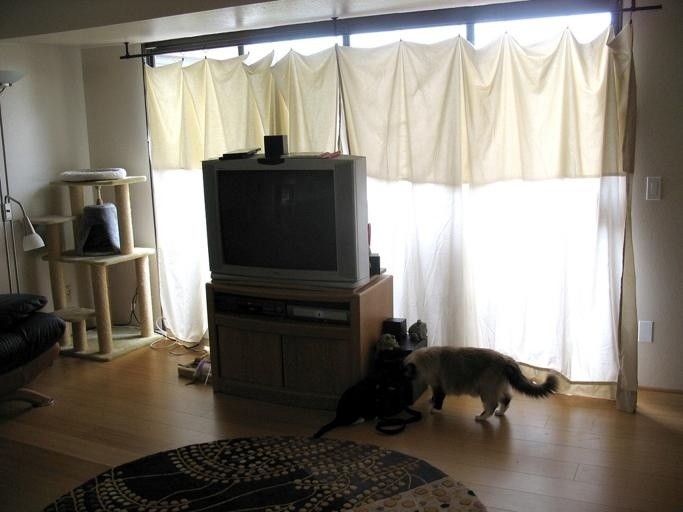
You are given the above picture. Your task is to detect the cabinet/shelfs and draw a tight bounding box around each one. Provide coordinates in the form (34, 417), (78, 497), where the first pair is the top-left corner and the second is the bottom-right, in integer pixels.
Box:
(204, 274), (392, 410)
(23, 169), (159, 360)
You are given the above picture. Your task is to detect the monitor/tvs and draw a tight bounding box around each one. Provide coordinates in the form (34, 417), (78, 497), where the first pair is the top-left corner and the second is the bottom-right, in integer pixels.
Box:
(202, 152), (370, 288)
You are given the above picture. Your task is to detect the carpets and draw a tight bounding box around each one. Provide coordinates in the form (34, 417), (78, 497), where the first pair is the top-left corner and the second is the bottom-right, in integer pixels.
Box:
(41, 435), (491, 512)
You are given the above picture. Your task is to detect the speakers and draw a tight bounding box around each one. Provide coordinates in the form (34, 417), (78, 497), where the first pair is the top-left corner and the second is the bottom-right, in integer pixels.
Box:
(370, 253), (380, 277)
(264, 135), (287, 156)
(384, 317), (407, 341)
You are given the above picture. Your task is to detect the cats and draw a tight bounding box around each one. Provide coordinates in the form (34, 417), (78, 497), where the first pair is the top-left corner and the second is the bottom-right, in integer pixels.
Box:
(313, 348), (414, 439)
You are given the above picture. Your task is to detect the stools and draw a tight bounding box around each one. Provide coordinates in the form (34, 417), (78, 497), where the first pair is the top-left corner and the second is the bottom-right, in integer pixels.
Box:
(0, 293), (65, 409)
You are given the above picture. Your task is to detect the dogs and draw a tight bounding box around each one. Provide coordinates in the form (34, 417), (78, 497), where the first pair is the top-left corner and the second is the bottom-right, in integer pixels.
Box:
(402, 346), (559, 421)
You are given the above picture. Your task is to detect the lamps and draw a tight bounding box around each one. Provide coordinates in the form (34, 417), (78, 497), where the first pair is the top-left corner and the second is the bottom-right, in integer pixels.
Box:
(0, 70), (46, 295)
